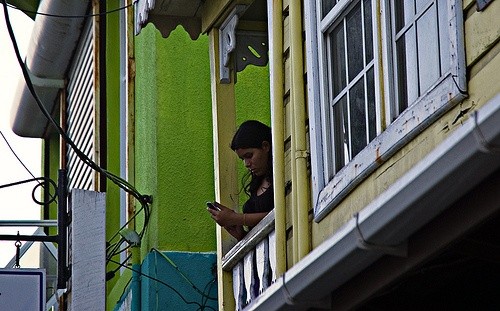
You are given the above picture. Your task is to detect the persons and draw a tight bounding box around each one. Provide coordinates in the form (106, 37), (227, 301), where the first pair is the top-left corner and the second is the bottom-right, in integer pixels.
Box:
(208, 119), (275, 240)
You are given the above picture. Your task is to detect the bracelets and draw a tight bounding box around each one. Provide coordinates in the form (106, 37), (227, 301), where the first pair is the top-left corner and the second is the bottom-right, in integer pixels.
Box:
(244, 213), (246, 226)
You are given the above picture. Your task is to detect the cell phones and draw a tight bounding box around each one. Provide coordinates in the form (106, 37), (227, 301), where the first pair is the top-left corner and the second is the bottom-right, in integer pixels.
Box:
(207, 202), (219, 211)
